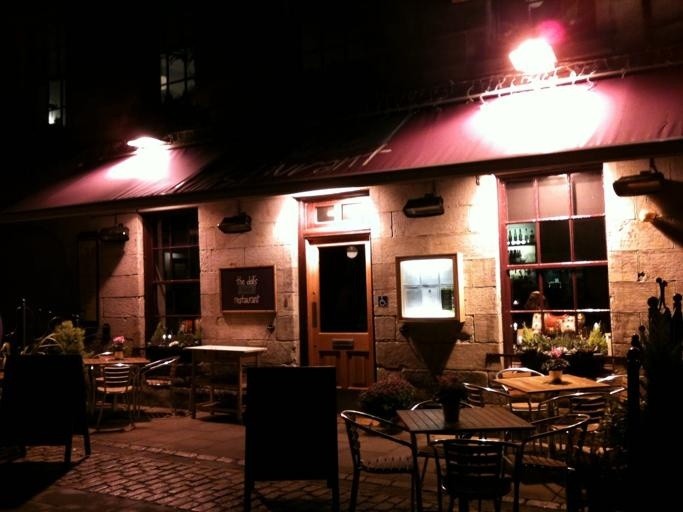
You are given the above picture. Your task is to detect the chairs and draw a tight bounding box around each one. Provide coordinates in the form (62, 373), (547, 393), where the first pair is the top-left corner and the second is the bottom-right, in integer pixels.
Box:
(81, 356), (180, 430)
(0, 354), (90, 462)
(244, 365), (339, 512)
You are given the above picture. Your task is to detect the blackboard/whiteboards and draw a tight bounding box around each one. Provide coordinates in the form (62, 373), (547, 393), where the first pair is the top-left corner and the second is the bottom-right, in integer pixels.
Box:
(218, 264), (278, 314)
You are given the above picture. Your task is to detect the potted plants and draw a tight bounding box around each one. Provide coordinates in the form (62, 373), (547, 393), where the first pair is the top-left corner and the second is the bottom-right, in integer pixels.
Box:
(519, 321), (607, 379)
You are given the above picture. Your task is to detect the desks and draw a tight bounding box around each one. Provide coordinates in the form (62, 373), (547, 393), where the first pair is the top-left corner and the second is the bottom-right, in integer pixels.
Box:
(183, 344), (268, 422)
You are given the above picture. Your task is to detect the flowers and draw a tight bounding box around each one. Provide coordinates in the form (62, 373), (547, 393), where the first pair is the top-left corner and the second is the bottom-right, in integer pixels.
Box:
(541, 347), (569, 370)
(112, 336), (128, 351)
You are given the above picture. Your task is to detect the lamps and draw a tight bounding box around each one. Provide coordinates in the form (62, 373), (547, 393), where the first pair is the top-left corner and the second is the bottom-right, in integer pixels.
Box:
(613, 174), (663, 197)
(99, 223), (129, 243)
(218, 213), (252, 233)
(403, 192), (444, 218)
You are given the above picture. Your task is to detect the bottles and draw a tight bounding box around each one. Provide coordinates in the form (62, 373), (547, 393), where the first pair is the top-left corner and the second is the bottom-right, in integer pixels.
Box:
(508, 249), (521, 264)
(508, 228), (535, 245)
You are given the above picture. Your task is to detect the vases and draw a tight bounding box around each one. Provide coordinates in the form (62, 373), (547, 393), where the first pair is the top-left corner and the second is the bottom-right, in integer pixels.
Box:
(115, 351), (124, 359)
